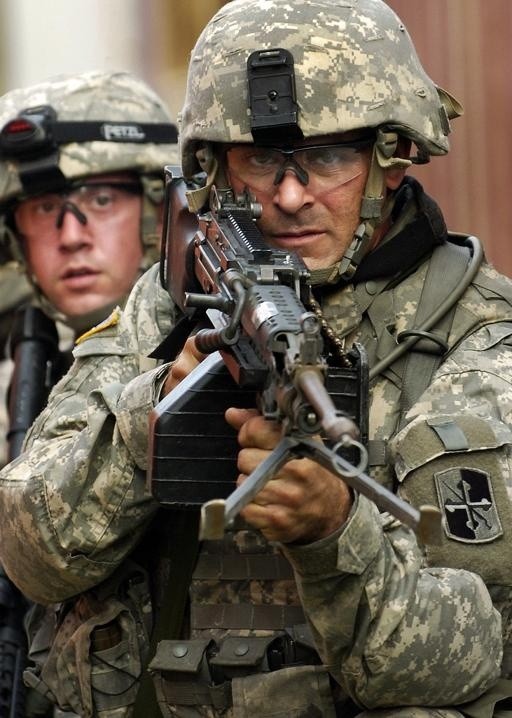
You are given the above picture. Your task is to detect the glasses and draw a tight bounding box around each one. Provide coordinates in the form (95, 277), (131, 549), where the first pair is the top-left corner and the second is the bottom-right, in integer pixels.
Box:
(225, 143), (373, 200)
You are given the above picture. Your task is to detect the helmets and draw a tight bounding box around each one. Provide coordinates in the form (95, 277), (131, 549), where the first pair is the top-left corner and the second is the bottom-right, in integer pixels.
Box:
(181, 0), (451, 181)
(14, 180), (139, 239)
(1, 67), (180, 206)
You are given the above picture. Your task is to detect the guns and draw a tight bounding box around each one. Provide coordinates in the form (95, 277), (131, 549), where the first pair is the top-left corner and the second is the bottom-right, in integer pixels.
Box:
(145, 164), (442, 547)
(6, 304), (59, 463)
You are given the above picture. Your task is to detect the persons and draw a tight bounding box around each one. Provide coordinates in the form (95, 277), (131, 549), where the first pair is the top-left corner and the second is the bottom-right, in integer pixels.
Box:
(1, 67), (181, 714)
(1, 0), (512, 715)
